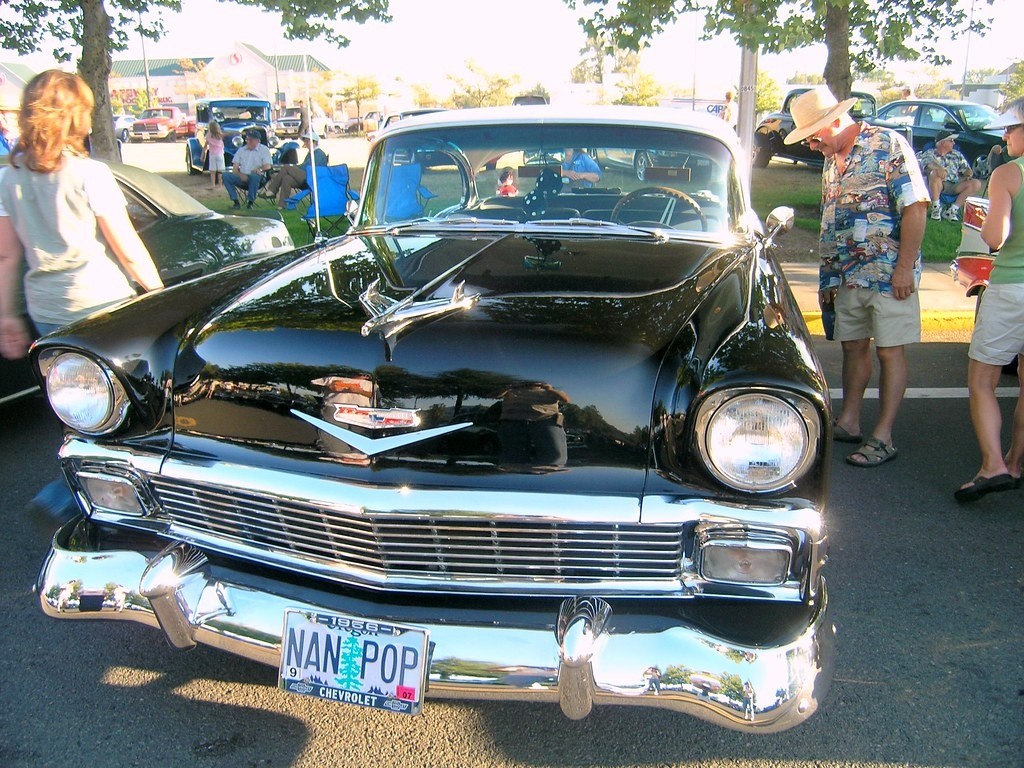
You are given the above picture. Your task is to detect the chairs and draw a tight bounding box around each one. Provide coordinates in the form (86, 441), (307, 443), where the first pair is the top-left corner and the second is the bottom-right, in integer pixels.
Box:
(462, 208), (580, 223)
(924, 141), (971, 221)
(234, 153), (360, 245)
(377, 161), (438, 222)
(584, 210), (715, 225)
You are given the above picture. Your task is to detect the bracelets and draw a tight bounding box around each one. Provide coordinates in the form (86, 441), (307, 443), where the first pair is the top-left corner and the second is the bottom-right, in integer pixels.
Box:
(258, 168), (263, 172)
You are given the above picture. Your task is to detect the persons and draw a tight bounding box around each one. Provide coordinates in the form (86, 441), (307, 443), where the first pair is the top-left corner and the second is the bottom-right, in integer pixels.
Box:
(258, 131), (327, 210)
(311, 372), (378, 464)
(496, 166), (519, 196)
(221, 129), (273, 209)
(955, 97), (1024, 498)
(500, 381), (569, 472)
(921, 130), (981, 222)
(901, 84), (918, 113)
(0, 69), (165, 523)
(724, 92), (738, 132)
(784, 87), (931, 466)
(200, 122), (225, 190)
(561, 148), (602, 188)
(297, 97), (318, 148)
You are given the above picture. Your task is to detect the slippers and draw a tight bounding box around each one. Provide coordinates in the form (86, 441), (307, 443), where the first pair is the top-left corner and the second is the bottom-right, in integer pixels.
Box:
(955, 473), (1024, 501)
(832, 418), (864, 444)
(847, 436), (898, 466)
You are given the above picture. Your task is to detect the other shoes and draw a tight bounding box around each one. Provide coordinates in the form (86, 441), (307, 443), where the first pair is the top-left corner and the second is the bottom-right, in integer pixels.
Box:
(247, 203), (253, 209)
(258, 193), (276, 198)
(230, 203), (241, 210)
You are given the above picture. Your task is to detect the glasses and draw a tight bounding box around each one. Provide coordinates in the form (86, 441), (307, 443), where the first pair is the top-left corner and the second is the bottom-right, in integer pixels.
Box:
(945, 138), (954, 142)
(1004, 124), (1024, 134)
(800, 129), (823, 147)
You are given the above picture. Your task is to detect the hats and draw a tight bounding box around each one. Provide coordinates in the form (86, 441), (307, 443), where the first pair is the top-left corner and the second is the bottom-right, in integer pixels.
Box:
(983, 110), (1024, 129)
(934, 131), (959, 145)
(783, 86), (858, 145)
(301, 132), (319, 142)
(245, 129), (262, 139)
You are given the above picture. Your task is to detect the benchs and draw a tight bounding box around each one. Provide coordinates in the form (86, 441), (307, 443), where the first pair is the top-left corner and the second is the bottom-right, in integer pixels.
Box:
(486, 195), (718, 218)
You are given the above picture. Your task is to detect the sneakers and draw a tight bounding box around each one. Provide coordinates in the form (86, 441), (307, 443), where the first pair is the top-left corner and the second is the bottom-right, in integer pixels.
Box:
(941, 208), (958, 224)
(931, 205), (943, 220)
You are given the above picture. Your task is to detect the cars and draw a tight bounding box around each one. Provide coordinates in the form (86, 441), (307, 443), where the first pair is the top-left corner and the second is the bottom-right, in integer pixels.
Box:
(271, 106), (503, 171)
(876, 97), (1024, 180)
(751, 83), (876, 172)
(0, 158), (296, 289)
(114, 102), (196, 142)
(185, 99), (276, 180)
(25, 107), (835, 735)
(585, 146), (716, 182)
